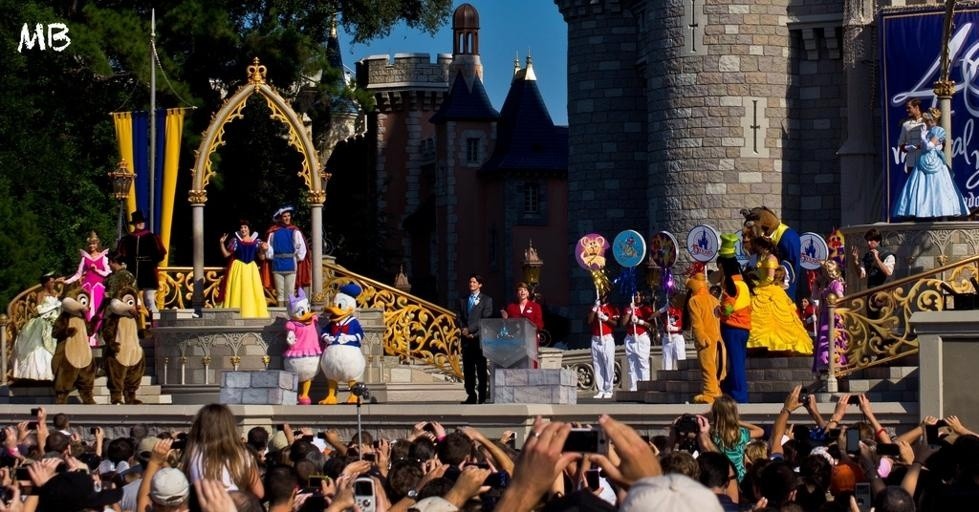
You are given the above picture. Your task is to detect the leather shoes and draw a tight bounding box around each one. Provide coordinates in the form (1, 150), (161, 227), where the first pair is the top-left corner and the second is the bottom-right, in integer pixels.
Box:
(461, 397), (485, 404)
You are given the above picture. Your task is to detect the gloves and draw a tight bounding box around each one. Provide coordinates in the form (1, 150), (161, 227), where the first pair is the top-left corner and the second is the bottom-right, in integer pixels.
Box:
(632, 315), (643, 326)
(597, 311), (608, 322)
(659, 303), (670, 313)
(626, 302), (635, 314)
(905, 145), (917, 151)
(920, 124), (929, 139)
(667, 324), (678, 332)
(592, 299), (601, 311)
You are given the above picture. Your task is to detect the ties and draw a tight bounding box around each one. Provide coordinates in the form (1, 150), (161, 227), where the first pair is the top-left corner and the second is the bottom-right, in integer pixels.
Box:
(468, 297), (475, 312)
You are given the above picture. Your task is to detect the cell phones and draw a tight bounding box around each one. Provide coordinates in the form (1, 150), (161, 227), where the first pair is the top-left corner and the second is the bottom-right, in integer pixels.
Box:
(585, 470), (600, 491)
(510, 432), (517, 440)
(561, 422), (610, 457)
(876, 443), (900, 456)
(847, 395), (860, 404)
(485, 471), (508, 488)
(846, 427), (861, 456)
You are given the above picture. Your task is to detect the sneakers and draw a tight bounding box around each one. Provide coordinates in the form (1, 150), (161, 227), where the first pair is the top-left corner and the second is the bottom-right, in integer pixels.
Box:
(593, 391), (613, 399)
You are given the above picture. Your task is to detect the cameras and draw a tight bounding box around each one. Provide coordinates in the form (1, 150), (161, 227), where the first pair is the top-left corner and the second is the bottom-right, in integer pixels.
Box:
(855, 482), (871, 512)
(863, 252), (875, 262)
(680, 414), (703, 434)
(799, 388), (808, 405)
(351, 382), (369, 399)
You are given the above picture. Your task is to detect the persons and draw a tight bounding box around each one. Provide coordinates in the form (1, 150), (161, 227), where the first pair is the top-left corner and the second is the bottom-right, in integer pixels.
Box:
(622, 289), (656, 391)
(220, 217), (273, 321)
(892, 107), (971, 221)
(97, 284), (148, 405)
(455, 273), (494, 404)
(586, 286), (622, 399)
(117, 210), (167, 330)
(812, 257), (851, 372)
(651, 290), (686, 371)
(59, 231), (112, 349)
(13, 267), (63, 383)
(897, 98), (943, 174)
(798, 296), (817, 340)
(500, 282), (544, 370)
(264, 205), (307, 307)
(52, 288), (101, 404)
(0, 381), (978, 512)
(746, 235), (812, 354)
(850, 228), (902, 361)
(95, 249), (137, 336)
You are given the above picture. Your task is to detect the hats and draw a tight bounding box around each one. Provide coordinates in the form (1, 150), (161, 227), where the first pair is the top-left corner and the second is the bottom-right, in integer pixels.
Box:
(621, 474), (725, 512)
(40, 471), (123, 512)
(150, 468), (188, 506)
(136, 437), (162, 460)
(830, 464), (866, 495)
